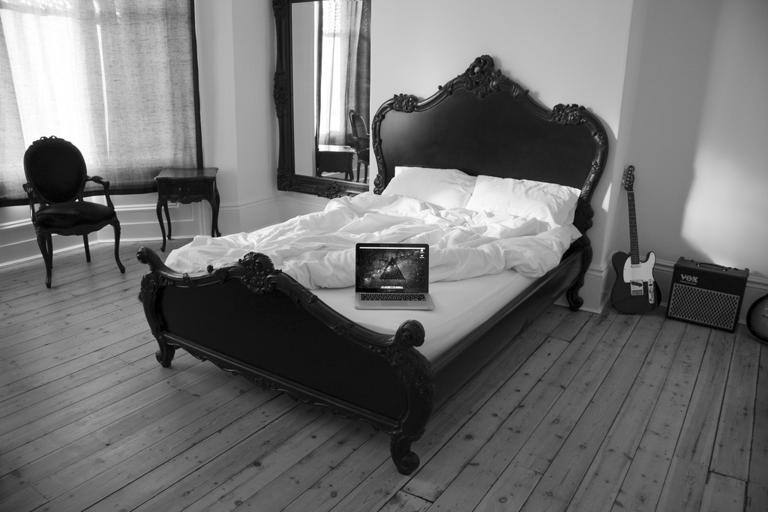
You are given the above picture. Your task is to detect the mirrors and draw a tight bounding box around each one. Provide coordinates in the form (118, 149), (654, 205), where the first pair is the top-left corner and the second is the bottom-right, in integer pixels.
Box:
(269, 1), (375, 199)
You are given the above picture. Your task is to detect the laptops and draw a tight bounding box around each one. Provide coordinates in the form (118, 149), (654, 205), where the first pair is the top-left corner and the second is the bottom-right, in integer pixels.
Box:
(355, 243), (435, 311)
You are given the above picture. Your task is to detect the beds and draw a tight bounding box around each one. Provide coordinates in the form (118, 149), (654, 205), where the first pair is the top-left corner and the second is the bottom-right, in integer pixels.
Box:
(132, 51), (609, 477)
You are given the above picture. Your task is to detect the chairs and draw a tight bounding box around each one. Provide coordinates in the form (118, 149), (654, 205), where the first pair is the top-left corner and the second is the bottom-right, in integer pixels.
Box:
(21, 135), (127, 289)
(347, 107), (371, 185)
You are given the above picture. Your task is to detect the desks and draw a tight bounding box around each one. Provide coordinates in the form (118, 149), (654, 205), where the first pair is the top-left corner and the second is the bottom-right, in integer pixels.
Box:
(154, 165), (223, 252)
(314, 143), (355, 184)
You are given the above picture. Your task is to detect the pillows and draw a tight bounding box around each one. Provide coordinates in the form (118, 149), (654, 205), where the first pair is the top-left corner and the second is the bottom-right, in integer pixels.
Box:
(462, 171), (582, 240)
(376, 163), (477, 214)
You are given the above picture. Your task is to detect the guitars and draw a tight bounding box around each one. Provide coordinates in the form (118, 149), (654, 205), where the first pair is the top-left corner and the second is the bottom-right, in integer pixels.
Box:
(611, 165), (662, 314)
